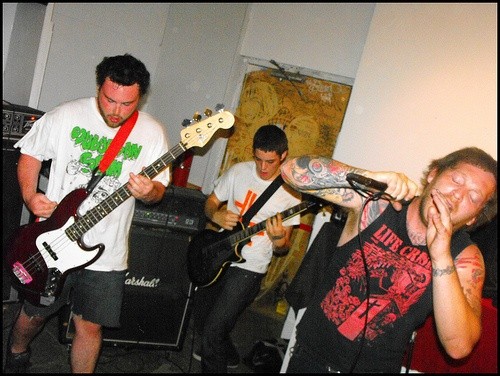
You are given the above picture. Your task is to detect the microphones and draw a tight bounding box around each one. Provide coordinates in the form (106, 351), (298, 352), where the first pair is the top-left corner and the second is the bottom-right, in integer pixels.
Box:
(347, 173), (412, 205)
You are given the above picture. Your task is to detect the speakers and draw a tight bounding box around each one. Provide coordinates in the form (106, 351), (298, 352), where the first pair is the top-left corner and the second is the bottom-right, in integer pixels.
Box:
(0, 137), (24, 301)
(58, 222), (199, 352)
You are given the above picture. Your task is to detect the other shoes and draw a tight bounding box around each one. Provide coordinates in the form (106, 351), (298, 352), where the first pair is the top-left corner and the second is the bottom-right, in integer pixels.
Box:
(9, 349), (30, 376)
(225, 344), (238, 369)
(193, 340), (202, 360)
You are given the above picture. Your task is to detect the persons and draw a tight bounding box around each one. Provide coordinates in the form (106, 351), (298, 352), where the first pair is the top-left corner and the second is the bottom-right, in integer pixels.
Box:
(190, 125), (299, 373)
(4, 54), (172, 373)
(279, 146), (496, 373)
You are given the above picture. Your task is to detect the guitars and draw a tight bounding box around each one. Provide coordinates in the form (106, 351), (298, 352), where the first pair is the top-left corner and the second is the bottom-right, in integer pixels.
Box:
(187, 197), (320, 288)
(2, 103), (236, 319)
